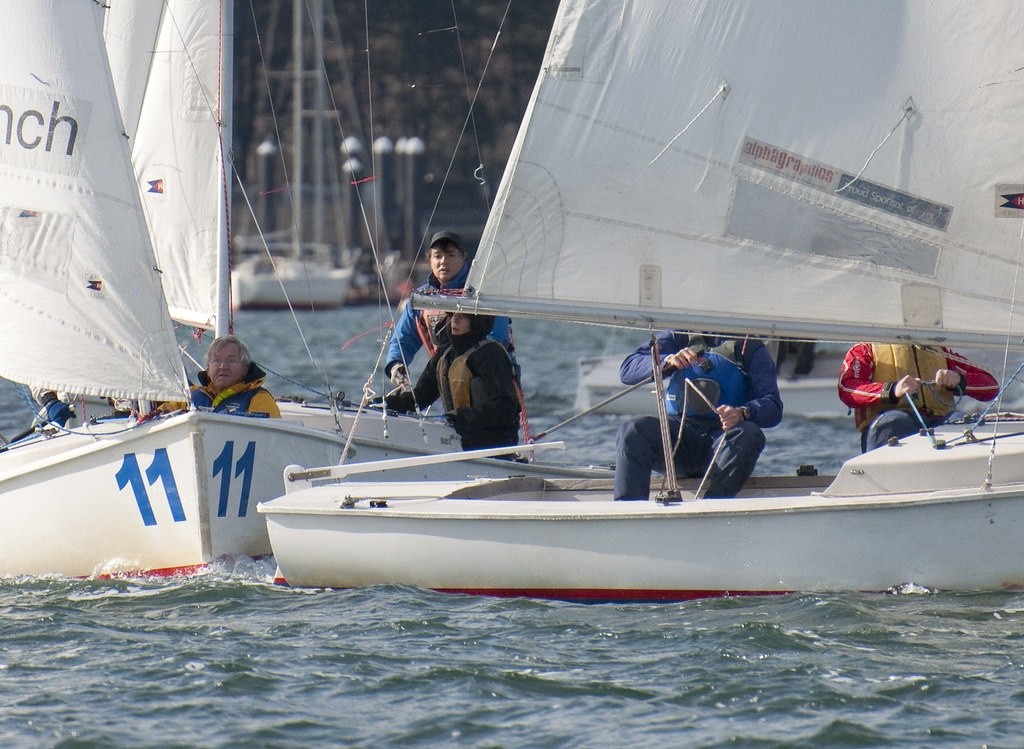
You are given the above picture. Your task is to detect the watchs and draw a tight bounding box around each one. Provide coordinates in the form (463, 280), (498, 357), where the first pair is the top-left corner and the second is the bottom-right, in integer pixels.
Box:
(740, 406), (750, 421)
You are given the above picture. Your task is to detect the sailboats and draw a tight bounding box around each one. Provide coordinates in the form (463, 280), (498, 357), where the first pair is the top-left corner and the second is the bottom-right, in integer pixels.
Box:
(231, 3), (363, 314)
(103, 0), (612, 477)
(248, 1), (1024, 603)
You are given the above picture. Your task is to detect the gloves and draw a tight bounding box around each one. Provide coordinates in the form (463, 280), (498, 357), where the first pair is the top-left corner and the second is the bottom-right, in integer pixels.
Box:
(41, 392), (77, 427)
(370, 395), (397, 410)
(443, 407), (465, 426)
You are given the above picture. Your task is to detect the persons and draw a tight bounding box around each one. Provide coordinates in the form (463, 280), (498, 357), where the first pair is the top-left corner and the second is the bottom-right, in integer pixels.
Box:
(838, 342), (1000, 454)
(614, 329), (783, 501)
(154, 335), (281, 419)
(384, 230), (520, 392)
(30, 385), (117, 431)
(366, 310), (522, 462)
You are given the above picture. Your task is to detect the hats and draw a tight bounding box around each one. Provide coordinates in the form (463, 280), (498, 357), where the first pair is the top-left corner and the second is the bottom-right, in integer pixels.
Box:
(428, 231), (463, 257)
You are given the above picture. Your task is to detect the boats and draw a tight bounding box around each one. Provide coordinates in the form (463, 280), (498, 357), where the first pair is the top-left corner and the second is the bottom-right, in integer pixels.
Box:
(0, 1), (468, 598)
(570, 345), (870, 424)
